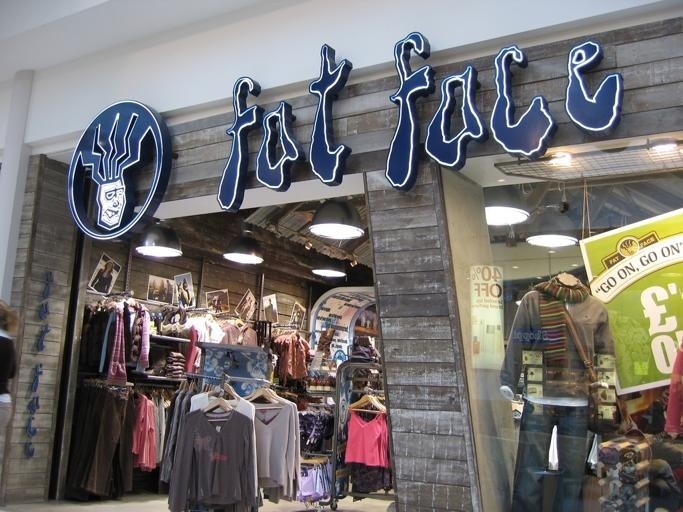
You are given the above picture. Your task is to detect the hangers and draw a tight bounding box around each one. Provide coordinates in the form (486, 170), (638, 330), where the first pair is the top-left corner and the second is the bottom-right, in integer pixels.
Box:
(84, 372), (281, 413)
(215, 313), (300, 335)
(348, 388), (386, 414)
(301, 452), (330, 470)
(97, 294), (209, 317)
(304, 402), (331, 413)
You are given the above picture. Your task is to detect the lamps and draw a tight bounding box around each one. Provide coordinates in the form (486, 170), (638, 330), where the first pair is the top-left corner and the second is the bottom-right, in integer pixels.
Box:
(485, 186), (530, 225)
(222, 223), (265, 264)
(526, 204), (578, 247)
(312, 256), (347, 277)
(308, 200), (365, 240)
(135, 218), (183, 258)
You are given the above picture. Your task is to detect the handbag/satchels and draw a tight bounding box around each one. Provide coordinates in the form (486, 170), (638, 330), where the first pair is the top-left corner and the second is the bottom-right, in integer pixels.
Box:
(588, 381), (622, 434)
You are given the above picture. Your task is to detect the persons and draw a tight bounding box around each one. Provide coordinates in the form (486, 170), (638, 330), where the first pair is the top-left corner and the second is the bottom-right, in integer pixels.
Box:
(499, 271), (618, 512)
(586, 384), (683, 511)
(0, 302), (21, 474)
